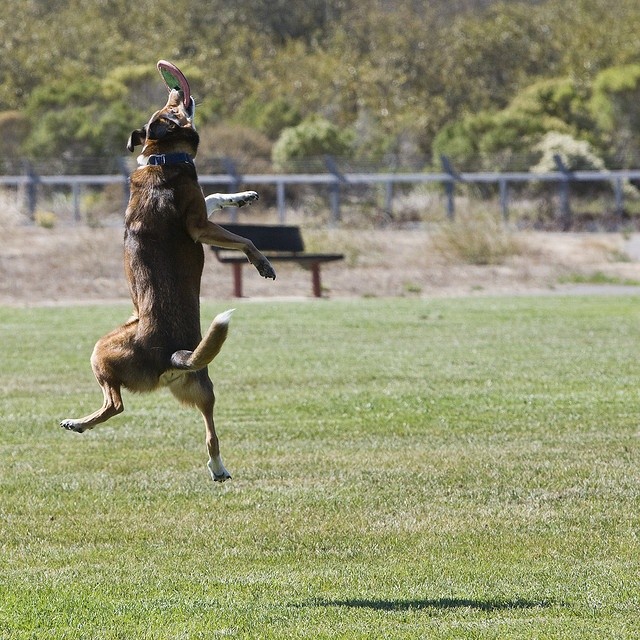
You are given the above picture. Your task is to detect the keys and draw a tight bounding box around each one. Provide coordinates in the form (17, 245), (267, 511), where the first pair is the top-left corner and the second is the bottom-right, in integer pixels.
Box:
(156, 60), (190, 109)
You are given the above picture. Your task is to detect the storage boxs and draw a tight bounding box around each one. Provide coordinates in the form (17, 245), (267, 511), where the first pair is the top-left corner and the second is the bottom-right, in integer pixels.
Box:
(208, 222), (347, 299)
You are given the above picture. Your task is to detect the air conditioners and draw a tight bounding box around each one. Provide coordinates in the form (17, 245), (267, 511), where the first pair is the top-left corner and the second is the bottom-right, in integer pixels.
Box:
(60, 85), (276, 481)
